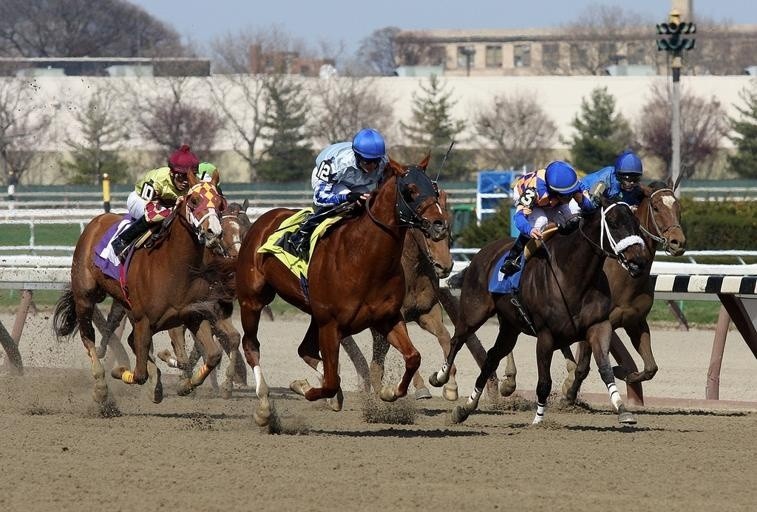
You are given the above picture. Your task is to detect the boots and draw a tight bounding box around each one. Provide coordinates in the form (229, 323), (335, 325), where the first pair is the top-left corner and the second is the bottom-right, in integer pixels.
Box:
(111, 215), (155, 265)
(500, 233), (531, 275)
(290, 206), (332, 263)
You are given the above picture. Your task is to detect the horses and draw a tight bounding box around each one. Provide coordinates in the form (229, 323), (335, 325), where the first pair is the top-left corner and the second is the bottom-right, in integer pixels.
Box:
(54, 167), (229, 404)
(235, 148), (451, 428)
(428, 185), (650, 427)
(100, 198), (247, 401)
(341, 187), (458, 401)
(500, 177), (687, 397)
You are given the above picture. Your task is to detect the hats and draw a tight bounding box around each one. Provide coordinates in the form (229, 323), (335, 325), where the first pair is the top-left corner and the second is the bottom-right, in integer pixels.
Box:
(545, 161), (580, 194)
(352, 128), (386, 158)
(169, 145), (199, 171)
(614, 152), (642, 175)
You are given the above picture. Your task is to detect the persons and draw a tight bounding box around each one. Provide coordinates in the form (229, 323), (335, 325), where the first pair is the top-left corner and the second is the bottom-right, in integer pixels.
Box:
(569, 150), (642, 220)
(500, 161), (596, 274)
(288, 128), (396, 262)
(110, 145), (199, 264)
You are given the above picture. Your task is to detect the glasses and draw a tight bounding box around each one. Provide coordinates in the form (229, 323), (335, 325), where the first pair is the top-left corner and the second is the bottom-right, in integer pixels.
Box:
(619, 174), (641, 182)
(360, 158), (381, 164)
(174, 173), (188, 182)
